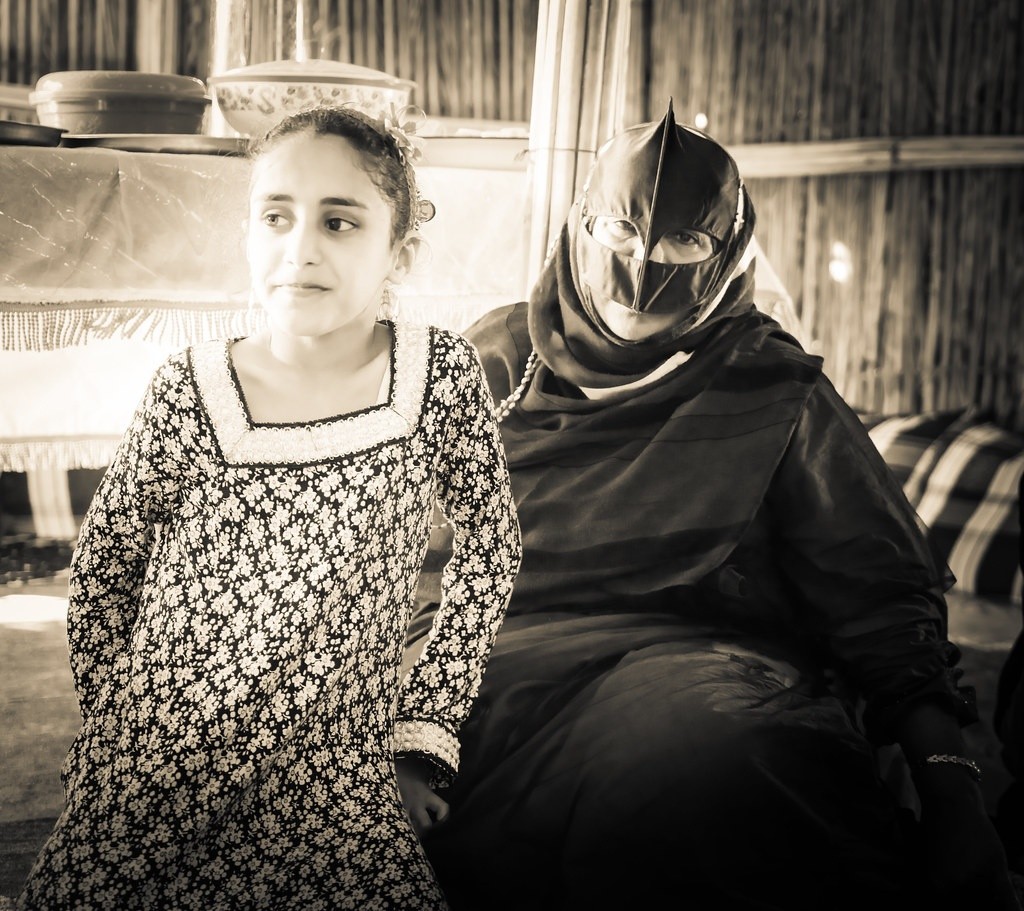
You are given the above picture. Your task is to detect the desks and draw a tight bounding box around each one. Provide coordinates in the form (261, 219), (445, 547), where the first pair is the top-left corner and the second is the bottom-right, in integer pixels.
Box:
(0, 139), (532, 545)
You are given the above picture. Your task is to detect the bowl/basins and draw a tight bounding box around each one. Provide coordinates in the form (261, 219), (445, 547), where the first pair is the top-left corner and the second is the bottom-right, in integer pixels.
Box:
(28, 71), (212, 135)
(206, 58), (418, 138)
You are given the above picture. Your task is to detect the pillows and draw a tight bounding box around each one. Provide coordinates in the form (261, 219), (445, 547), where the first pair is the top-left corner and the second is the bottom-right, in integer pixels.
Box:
(855, 407), (1024, 609)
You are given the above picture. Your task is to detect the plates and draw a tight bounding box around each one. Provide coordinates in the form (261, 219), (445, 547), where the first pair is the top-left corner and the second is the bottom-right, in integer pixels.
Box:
(0, 119), (69, 148)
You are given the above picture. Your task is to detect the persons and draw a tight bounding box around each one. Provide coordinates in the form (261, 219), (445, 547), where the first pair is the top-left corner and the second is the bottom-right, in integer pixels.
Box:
(401, 95), (1024, 911)
(12, 106), (524, 911)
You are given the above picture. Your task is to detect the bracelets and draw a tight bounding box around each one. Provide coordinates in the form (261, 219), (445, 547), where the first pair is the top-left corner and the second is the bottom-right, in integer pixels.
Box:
(926, 754), (984, 784)
